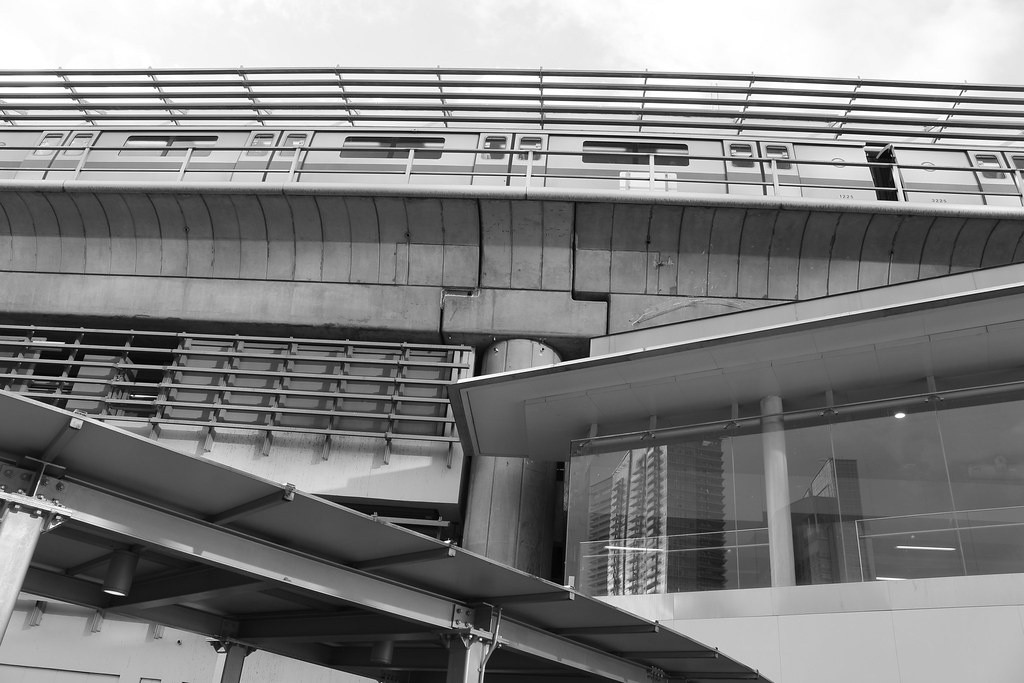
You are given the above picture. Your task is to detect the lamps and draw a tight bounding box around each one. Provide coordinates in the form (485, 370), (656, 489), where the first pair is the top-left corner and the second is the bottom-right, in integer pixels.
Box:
(101, 548), (139, 597)
(370, 640), (394, 664)
